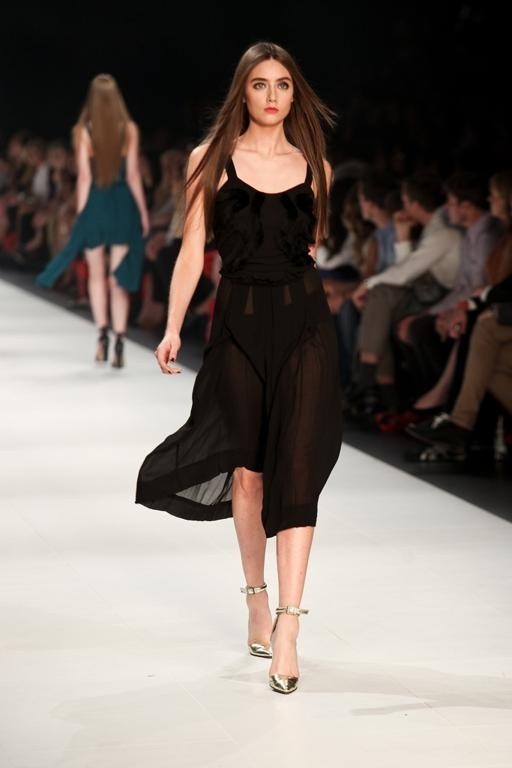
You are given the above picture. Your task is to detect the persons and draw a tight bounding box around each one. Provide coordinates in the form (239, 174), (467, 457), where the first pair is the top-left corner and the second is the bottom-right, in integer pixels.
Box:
(1, 128), (225, 340)
(312, 156), (510, 467)
(155, 40), (346, 696)
(70, 72), (152, 368)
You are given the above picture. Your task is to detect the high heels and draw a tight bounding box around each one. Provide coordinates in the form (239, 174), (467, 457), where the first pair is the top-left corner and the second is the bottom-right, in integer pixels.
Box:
(240, 584), (309, 694)
(96, 326), (126, 367)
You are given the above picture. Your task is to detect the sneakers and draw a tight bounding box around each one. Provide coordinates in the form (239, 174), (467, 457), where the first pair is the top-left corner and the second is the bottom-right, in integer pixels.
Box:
(344, 361), (473, 464)
